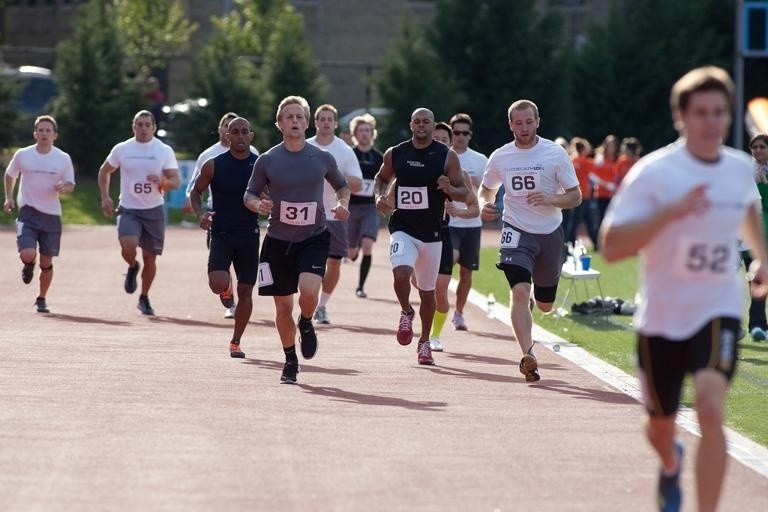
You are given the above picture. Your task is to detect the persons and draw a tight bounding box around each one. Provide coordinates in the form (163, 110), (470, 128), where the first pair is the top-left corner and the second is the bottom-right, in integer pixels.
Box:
(384, 122), (480, 351)
(97, 109), (182, 316)
(596, 63), (768, 512)
(338, 127), (355, 149)
(3, 115), (76, 314)
(447, 112), (489, 331)
(243, 95), (351, 384)
(304, 103), (366, 324)
(735, 134), (768, 343)
(185, 111), (259, 318)
(587, 135), (620, 228)
(372, 107), (470, 366)
(477, 99), (583, 382)
(188, 116), (270, 359)
(144, 76), (165, 138)
(613, 137), (642, 193)
(345, 113), (385, 299)
(563, 137), (617, 255)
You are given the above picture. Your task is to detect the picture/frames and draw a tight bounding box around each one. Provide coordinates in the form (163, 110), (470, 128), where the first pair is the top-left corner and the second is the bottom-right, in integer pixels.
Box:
(556, 261), (609, 323)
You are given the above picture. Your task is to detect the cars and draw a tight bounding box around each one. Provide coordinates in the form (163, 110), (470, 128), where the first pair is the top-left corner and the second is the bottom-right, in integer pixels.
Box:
(580, 255), (591, 271)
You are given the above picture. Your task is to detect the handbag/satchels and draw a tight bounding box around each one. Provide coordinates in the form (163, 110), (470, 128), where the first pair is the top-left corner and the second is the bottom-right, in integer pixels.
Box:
(336, 198), (349, 205)
(159, 177), (167, 191)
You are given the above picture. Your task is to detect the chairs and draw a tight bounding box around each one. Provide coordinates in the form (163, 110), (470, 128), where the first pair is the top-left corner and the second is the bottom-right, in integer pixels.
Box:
(224, 304), (236, 318)
(280, 359), (299, 384)
(520, 352), (540, 382)
(125, 261), (140, 293)
(417, 341), (434, 364)
(137, 295), (153, 314)
(751, 327), (766, 342)
(220, 289), (234, 308)
(36, 297), (49, 312)
(429, 338), (443, 351)
(397, 305), (415, 346)
(298, 315), (319, 360)
(22, 263), (35, 284)
(452, 312), (467, 330)
(230, 338), (245, 358)
(314, 306), (329, 323)
(356, 289), (366, 298)
(657, 443), (682, 511)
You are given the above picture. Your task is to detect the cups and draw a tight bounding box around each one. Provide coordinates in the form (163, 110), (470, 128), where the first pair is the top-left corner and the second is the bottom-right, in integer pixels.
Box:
(453, 130), (472, 136)
